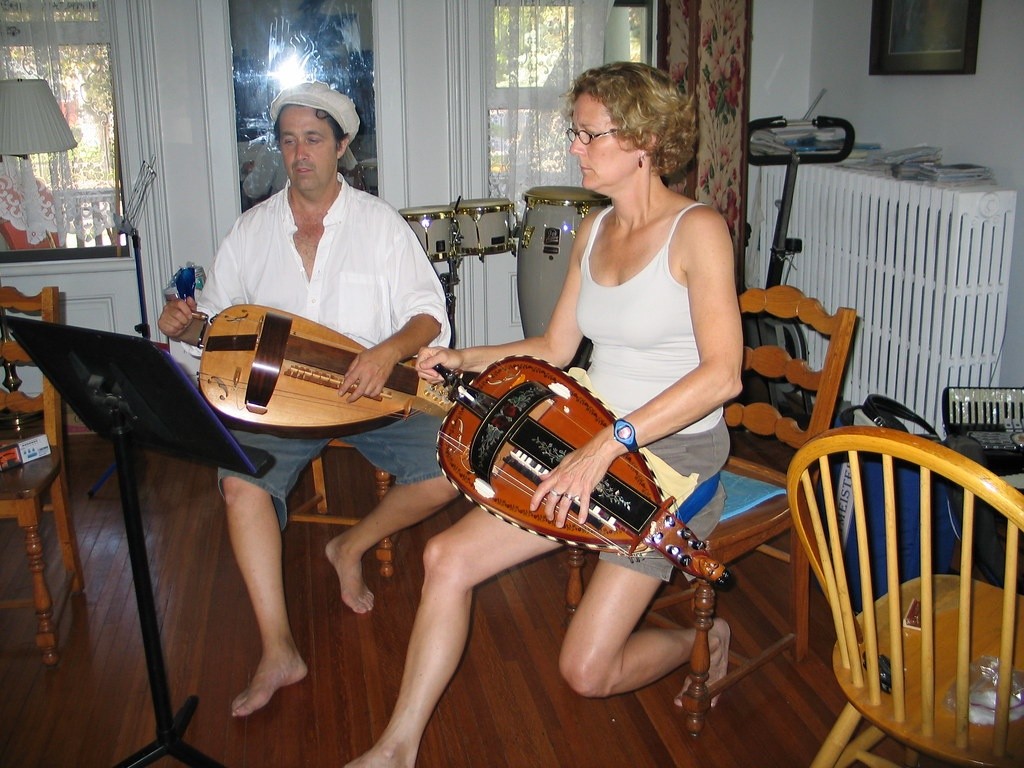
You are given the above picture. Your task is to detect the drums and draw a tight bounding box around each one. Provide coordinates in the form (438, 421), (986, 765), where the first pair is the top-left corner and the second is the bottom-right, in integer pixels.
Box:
(516, 185), (613, 339)
(398, 197), (512, 263)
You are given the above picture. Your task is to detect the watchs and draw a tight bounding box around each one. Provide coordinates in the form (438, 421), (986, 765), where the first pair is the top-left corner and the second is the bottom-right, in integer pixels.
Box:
(613, 418), (639, 452)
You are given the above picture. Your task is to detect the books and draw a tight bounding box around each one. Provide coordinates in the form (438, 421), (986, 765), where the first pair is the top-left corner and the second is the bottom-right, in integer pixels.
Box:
(752, 118), (989, 183)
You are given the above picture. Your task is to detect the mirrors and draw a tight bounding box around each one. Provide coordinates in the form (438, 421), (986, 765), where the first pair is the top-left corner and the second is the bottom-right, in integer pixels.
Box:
(196, 0), (409, 256)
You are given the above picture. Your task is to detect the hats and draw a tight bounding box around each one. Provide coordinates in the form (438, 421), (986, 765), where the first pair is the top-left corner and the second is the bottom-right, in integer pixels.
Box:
(269, 80), (361, 147)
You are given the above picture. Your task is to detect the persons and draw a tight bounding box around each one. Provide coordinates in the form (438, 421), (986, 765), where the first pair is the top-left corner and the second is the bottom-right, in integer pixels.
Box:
(342, 61), (742, 767)
(158, 80), (461, 716)
(239, 128), (360, 198)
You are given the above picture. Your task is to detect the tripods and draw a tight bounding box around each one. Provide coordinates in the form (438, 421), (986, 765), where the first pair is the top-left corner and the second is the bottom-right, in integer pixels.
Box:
(0, 309), (274, 768)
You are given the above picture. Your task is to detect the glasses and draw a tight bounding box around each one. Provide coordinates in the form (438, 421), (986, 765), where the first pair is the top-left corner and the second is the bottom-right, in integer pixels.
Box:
(566, 127), (616, 144)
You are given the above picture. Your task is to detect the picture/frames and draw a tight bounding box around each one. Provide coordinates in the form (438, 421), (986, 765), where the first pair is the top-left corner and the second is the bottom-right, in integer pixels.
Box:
(869, 0), (983, 75)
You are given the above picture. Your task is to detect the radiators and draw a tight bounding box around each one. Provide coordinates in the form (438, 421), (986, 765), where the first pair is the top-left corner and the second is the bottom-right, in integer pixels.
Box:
(758, 164), (1017, 443)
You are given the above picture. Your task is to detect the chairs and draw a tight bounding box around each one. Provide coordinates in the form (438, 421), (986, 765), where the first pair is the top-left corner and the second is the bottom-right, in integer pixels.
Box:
(0, 285), (84, 664)
(564, 287), (855, 738)
(787, 427), (1024, 768)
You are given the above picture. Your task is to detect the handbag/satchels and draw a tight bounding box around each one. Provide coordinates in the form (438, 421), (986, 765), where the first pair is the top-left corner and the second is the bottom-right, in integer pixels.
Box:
(809, 391), (962, 613)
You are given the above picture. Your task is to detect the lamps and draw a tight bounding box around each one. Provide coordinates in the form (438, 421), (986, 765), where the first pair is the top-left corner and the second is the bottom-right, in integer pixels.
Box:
(0, 78), (77, 243)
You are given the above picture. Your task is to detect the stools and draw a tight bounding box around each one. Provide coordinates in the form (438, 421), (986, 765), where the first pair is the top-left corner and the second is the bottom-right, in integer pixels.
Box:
(287, 439), (399, 575)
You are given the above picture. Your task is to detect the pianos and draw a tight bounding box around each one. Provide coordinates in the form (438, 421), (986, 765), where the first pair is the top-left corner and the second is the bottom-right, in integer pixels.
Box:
(943, 387), (1023, 468)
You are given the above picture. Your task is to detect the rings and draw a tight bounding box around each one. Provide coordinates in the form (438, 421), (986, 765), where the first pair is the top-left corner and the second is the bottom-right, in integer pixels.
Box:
(551, 490), (561, 496)
(564, 492), (575, 501)
